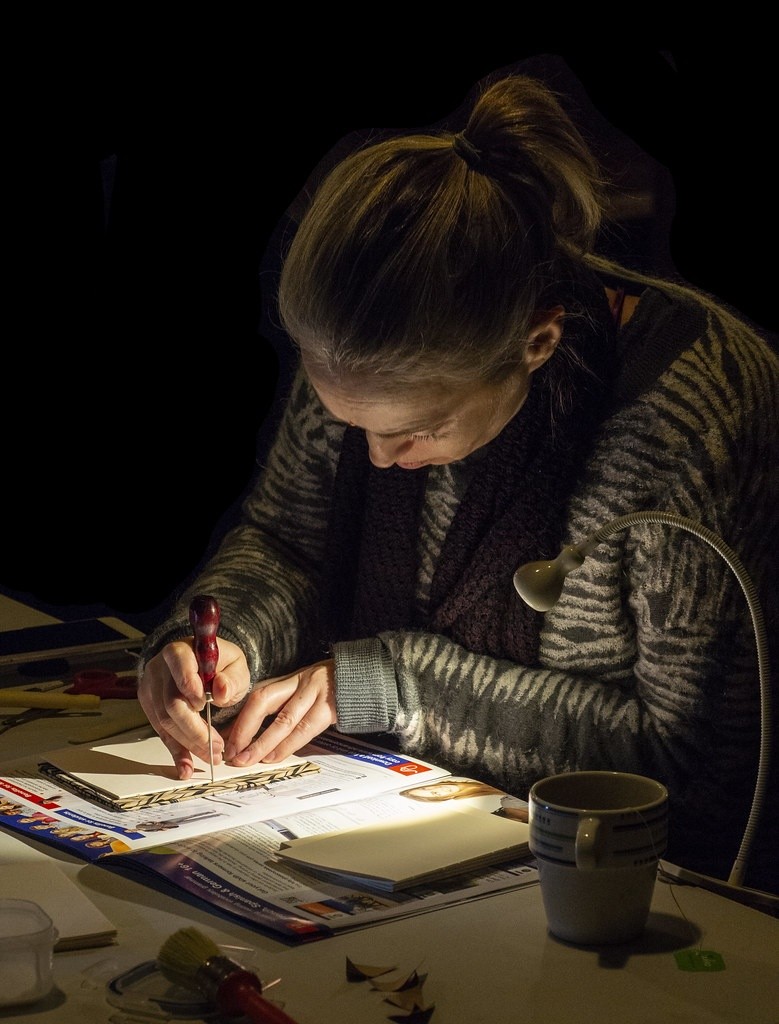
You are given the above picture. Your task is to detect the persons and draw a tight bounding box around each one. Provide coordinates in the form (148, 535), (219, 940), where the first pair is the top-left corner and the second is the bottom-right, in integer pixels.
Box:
(136, 72), (778, 897)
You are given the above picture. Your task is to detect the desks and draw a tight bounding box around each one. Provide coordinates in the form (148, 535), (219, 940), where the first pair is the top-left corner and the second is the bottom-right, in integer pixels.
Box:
(0, 590), (777, 1024)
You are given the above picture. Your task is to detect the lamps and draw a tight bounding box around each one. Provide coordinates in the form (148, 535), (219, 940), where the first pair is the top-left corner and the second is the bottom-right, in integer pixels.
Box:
(513, 508), (769, 890)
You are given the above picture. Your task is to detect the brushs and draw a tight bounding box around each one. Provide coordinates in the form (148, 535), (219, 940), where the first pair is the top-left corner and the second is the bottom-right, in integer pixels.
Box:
(156, 924), (303, 1024)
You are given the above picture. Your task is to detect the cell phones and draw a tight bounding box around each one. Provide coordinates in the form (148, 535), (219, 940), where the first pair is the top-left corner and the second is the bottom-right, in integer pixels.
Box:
(0, 616), (146, 667)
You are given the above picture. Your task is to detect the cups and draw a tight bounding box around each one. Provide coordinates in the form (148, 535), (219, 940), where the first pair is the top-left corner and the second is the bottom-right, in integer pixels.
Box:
(527, 772), (670, 946)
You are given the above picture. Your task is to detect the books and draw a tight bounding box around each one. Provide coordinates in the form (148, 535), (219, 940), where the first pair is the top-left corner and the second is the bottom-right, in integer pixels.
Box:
(0, 721), (541, 948)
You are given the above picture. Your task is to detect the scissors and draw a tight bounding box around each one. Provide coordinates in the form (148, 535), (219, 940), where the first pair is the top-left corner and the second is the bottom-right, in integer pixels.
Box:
(3, 666), (143, 746)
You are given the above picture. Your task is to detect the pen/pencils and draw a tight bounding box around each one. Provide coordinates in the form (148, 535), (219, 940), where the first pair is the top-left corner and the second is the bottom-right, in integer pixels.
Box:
(0, 688), (101, 712)
